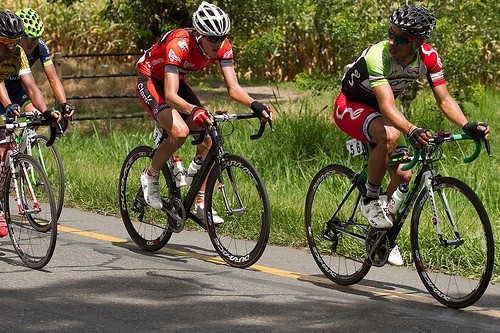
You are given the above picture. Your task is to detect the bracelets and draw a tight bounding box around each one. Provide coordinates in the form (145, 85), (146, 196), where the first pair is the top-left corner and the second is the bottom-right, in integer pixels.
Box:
(407, 124), (413, 136)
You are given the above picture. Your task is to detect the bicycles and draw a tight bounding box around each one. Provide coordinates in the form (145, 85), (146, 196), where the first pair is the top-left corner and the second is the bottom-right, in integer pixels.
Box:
(0, 106), (72, 269)
(304, 123), (494, 306)
(119, 104), (272, 269)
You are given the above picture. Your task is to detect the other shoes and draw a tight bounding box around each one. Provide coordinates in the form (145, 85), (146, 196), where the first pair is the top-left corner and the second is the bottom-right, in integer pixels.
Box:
(0, 216), (8, 236)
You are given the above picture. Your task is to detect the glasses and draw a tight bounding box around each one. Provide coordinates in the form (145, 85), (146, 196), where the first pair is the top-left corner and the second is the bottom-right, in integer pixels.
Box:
(205, 35), (227, 43)
(2, 38), (20, 45)
(388, 26), (415, 45)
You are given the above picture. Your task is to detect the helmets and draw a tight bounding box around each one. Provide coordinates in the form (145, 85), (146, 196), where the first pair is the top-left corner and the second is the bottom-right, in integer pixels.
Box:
(15, 8), (45, 39)
(192, 1), (231, 36)
(389, 5), (437, 40)
(0, 10), (25, 38)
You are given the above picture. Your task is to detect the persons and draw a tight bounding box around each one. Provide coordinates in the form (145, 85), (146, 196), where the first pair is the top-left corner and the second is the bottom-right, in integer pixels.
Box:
(334, 5), (492, 266)
(134, 1), (274, 223)
(0, 8), (74, 240)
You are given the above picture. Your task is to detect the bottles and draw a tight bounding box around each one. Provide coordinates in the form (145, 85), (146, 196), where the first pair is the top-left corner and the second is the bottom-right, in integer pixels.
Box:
(386, 182), (409, 214)
(379, 188), (393, 223)
(173, 156), (188, 187)
(185, 154), (203, 185)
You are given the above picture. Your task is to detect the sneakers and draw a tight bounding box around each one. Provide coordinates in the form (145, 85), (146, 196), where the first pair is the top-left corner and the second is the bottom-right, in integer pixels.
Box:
(361, 197), (393, 228)
(140, 169), (163, 210)
(192, 204), (224, 224)
(387, 244), (403, 266)
